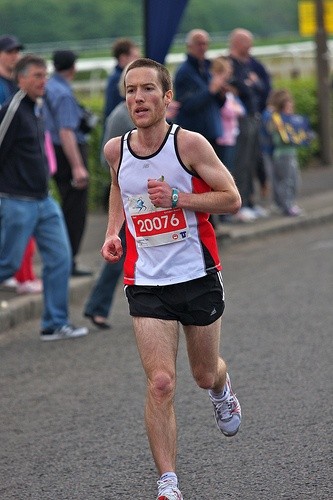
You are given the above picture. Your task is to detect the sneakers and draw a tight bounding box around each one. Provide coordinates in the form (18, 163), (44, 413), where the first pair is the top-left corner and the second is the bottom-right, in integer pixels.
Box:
(39, 321), (88, 341)
(155, 478), (183, 500)
(208, 372), (242, 437)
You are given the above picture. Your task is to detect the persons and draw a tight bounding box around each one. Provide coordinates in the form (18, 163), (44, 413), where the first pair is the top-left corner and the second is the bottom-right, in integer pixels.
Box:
(0, 130), (53, 294)
(101, 58), (242, 500)
(0, 35), (28, 290)
(81, 61), (140, 332)
(43, 49), (95, 277)
(0, 53), (91, 342)
(99, 27), (310, 238)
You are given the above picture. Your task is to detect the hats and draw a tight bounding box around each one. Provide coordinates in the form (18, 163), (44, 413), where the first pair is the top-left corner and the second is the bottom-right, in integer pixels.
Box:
(53, 50), (77, 70)
(0, 37), (25, 51)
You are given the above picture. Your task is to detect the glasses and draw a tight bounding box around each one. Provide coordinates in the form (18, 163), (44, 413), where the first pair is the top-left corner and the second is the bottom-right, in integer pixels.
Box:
(27, 72), (49, 80)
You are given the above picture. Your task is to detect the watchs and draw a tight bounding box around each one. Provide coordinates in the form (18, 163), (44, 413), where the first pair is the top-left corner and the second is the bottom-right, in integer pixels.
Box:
(172, 189), (179, 208)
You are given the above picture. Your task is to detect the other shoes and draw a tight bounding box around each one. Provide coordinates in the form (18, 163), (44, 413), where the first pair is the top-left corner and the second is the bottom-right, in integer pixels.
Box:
(231, 207), (257, 223)
(16, 279), (44, 294)
(286, 206), (304, 216)
(255, 204), (269, 218)
(73, 270), (92, 276)
(84, 312), (111, 329)
(2, 277), (18, 288)
